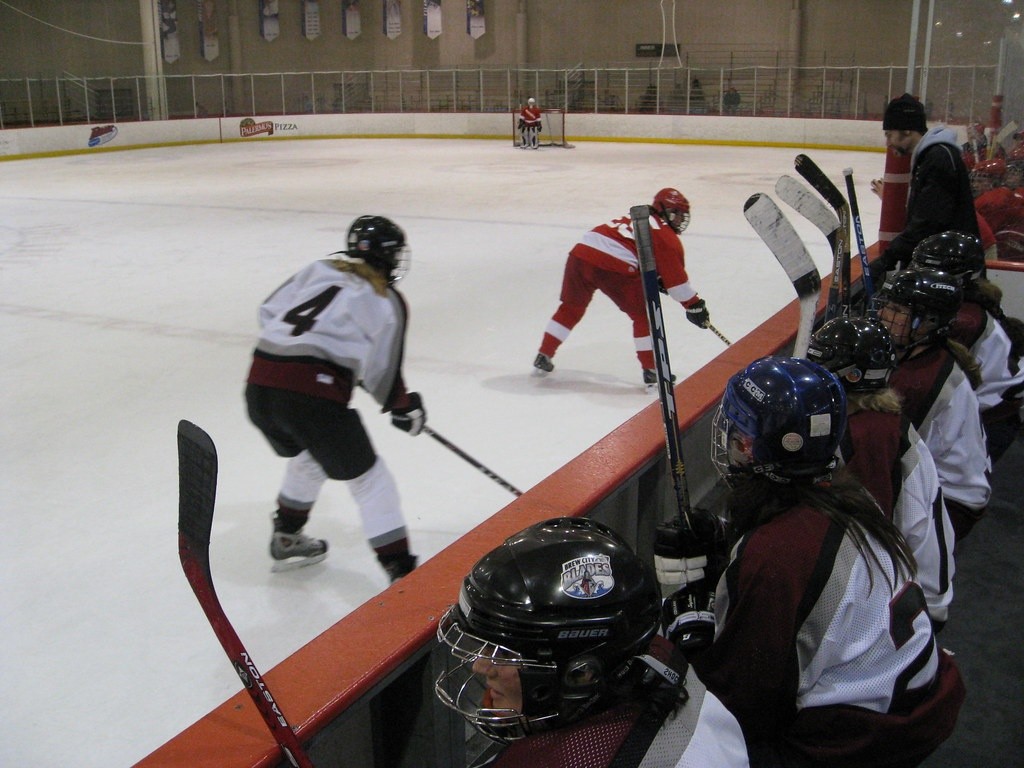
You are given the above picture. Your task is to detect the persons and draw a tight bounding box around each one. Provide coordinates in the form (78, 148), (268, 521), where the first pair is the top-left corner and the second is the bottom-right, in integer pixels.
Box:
(518, 97), (542, 150)
(960, 94), (1024, 262)
(244, 214), (428, 586)
(429, 518), (755, 766)
(723, 85), (741, 115)
(657, 352), (967, 767)
(910, 231), (1024, 420)
(868, 92), (987, 283)
(690, 78), (706, 112)
(640, 86), (663, 112)
(810, 314), (958, 627)
(532, 187), (710, 395)
(868, 262), (993, 539)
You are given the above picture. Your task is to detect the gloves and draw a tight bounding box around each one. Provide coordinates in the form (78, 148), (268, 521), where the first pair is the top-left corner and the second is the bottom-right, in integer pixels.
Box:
(661, 583), (715, 658)
(653, 507), (734, 585)
(391, 392), (426, 436)
(686, 299), (710, 329)
(656, 276), (669, 295)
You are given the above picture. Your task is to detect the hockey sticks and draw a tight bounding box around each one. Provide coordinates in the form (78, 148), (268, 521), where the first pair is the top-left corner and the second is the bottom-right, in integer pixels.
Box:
(704, 319), (732, 347)
(630, 204), (691, 515)
(843, 167), (879, 317)
(743, 192), (822, 359)
(360, 379), (524, 498)
(794, 153), (852, 318)
(775, 175), (845, 325)
(177, 419), (316, 768)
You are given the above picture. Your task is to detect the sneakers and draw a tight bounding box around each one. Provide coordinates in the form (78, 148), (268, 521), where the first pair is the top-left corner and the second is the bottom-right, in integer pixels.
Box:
(268, 517), (330, 572)
(378, 552), (417, 583)
(533, 353), (554, 375)
(643, 368), (676, 388)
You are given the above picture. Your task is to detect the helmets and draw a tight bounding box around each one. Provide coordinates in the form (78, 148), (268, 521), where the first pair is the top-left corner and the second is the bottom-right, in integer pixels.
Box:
(652, 188), (691, 234)
(866, 266), (963, 351)
(348, 215), (410, 285)
(528, 98), (535, 109)
(907, 230), (985, 289)
(806, 317), (897, 391)
(710, 356), (846, 495)
(965, 119), (1024, 197)
(433, 517), (663, 747)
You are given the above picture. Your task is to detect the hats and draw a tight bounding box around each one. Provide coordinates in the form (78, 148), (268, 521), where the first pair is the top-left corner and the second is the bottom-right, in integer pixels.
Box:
(882, 93), (926, 131)
